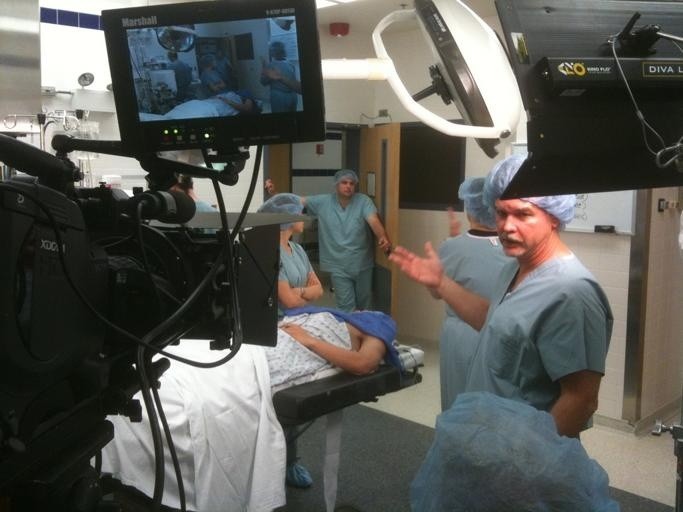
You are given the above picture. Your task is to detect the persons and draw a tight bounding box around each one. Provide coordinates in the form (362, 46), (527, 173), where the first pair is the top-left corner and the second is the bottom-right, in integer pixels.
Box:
(247, 191), (325, 490)
(168, 175), (222, 234)
(415, 387), (628, 509)
(217, 88), (255, 112)
(196, 53), (226, 95)
(265, 69), (301, 94)
(258, 306), (398, 397)
(389, 154), (613, 436)
(260, 42), (297, 113)
(214, 48), (233, 82)
(266, 167), (391, 308)
(165, 51), (188, 91)
(430, 172), (518, 410)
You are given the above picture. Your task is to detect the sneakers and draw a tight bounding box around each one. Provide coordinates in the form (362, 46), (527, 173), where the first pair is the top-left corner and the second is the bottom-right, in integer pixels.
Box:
(285, 464), (312, 487)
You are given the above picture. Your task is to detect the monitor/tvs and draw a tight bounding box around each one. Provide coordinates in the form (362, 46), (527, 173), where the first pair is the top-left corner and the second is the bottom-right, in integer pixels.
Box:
(374, 118), (466, 212)
(100, 1), (327, 153)
(497, 0), (682, 202)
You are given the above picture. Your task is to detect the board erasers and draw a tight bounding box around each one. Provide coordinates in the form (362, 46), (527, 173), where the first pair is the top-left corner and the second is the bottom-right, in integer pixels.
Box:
(594, 226), (615, 233)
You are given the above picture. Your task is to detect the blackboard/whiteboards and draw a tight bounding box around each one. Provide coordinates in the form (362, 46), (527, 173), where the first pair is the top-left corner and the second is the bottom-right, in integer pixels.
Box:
(465, 120), (638, 237)
